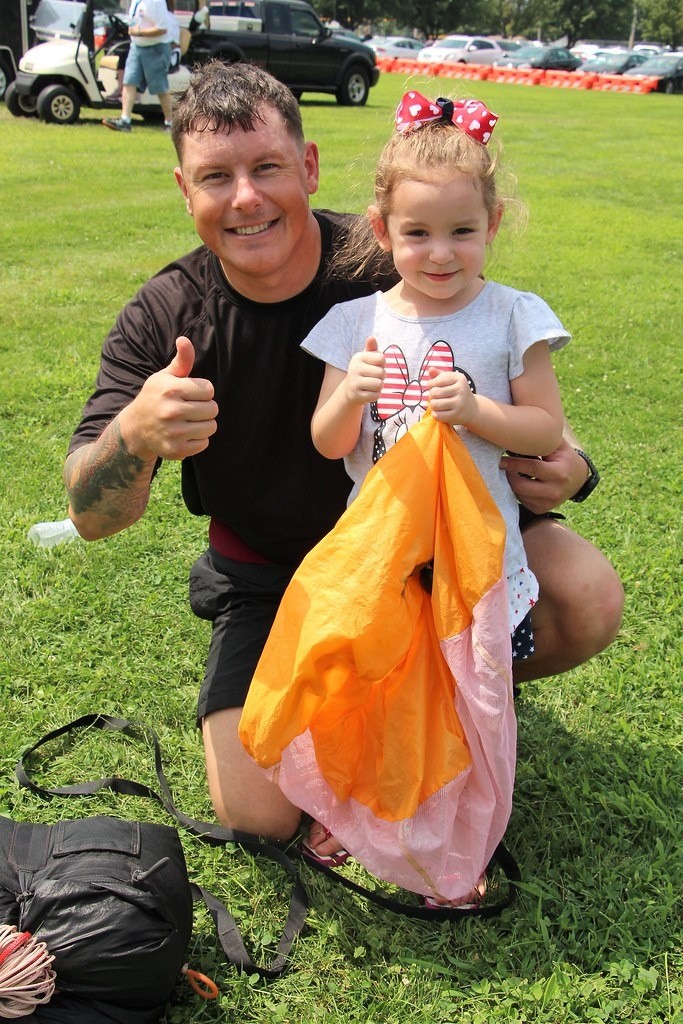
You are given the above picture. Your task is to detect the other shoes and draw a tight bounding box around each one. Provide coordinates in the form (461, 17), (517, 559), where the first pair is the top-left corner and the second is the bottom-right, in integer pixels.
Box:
(107, 88), (122, 100)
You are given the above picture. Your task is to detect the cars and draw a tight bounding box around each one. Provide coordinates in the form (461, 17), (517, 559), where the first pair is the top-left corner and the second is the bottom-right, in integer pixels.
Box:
(622, 55), (683, 95)
(576, 52), (648, 77)
(488, 35), (683, 61)
(494, 45), (582, 73)
(364, 34), (428, 60)
(416, 36), (503, 68)
(327, 27), (372, 44)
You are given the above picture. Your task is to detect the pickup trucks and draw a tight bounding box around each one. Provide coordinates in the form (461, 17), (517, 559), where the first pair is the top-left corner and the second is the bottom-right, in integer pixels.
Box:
(178, 1), (380, 107)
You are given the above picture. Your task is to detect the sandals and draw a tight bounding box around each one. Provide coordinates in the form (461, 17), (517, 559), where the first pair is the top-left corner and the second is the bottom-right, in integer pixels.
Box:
(301, 821), (350, 867)
(425, 865), (488, 910)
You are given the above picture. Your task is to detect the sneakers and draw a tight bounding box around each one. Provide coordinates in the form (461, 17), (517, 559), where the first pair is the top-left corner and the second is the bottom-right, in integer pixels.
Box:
(102, 118), (132, 132)
(162, 125), (172, 132)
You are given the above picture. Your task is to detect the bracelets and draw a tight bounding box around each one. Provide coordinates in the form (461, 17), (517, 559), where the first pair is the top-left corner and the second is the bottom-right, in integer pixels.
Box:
(136, 30), (141, 36)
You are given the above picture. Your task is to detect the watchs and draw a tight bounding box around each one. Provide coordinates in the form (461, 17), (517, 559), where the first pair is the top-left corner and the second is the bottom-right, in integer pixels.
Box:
(569, 449), (600, 503)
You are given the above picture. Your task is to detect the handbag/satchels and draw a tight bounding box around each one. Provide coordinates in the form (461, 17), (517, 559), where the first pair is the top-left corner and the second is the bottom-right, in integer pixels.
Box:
(0, 814), (191, 1024)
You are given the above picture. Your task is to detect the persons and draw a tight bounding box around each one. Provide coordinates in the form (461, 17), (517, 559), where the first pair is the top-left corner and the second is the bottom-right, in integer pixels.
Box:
(103, 0), (180, 133)
(65, 64), (625, 842)
(297, 93), (570, 913)
